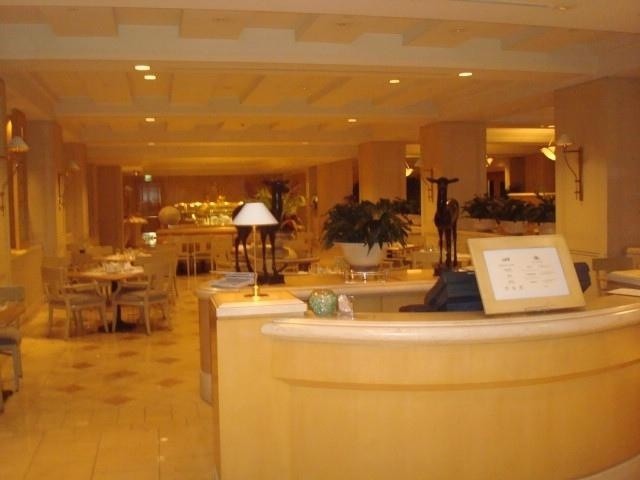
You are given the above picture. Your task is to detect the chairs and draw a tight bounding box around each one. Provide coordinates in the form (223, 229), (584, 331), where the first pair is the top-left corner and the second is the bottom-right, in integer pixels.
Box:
(193, 238), (214, 275)
(63, 232), (98, 268)
(40, 256), (109, 341)
(111, 254), (180, 335)
(592, 258), (633, 297)
(176, 237), (190, 276)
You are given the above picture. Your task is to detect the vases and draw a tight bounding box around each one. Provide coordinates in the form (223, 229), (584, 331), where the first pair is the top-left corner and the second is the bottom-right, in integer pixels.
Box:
(123, 217), (148, 248)
(141, 232), (157, 248)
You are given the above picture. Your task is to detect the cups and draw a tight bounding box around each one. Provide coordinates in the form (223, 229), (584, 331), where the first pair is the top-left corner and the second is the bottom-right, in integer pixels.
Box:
(333, 284), (355, 321)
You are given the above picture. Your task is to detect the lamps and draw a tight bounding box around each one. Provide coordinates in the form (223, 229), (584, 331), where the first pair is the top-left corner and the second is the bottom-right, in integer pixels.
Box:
(406, 161), (413, 176)
(0, 136), (29, 167)
(47, 152), (80, 176)
(542, 130), (556, 162)
(414, 154), (437, 202)
(486, 154), (493, 167)
(556, 132), (583, 201)
(145, 170), (152, 183)
(158, 206), (181, 225)
(231, 203), (279, 297)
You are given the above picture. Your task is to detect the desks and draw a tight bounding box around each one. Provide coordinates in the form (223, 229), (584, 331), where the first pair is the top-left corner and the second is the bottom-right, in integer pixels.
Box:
(607, 269), (640, 296)
(156, 227), (238, 271)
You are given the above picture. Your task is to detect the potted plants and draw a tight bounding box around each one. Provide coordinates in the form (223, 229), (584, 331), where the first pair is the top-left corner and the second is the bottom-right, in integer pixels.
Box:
(244, 175), (306, 273)
(321, 194), (413, 271)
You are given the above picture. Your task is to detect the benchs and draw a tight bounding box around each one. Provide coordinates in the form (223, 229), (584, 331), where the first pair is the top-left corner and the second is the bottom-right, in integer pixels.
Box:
(0, 290), (71, 393)
